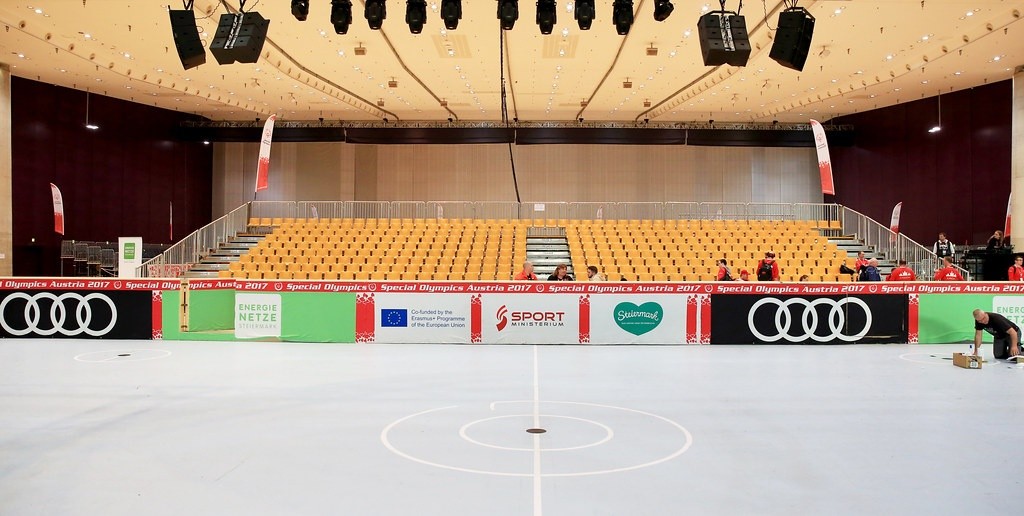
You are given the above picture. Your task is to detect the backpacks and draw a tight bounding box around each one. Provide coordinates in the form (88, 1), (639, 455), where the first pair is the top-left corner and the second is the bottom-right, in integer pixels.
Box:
(723, 267), (735, 281)
(758, 259), (775, 281)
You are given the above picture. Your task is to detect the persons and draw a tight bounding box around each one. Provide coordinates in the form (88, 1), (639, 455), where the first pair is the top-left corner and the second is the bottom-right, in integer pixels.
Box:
(1008, 256), (1024, 281)
(587, 266), (606, 281)
(547, 264), (573, 281)
(987, 230), (1004, 248)
(717, 259), (727, 281)
(855, 251), (884, 281)
(886, 259), (916, 281)
(515, 262), (538, 280)
(933, 232), (955, 262)
(621, 278), (627, 281)
(972, 309), (1024, 359)
(734, 269), (751, 281)
(934, 256), (964, 281)
(756, 251), (781, 282)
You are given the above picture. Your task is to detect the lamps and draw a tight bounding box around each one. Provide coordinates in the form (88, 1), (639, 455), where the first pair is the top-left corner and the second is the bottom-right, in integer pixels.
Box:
(440, 98), (448, 107)
(495, 0), (519, 30)
(623, 78), (632, 88)
(581, 99), (588, 107)
(769, 0), (815, 72)
(406, 0), (427, 34)
(644, 99), (650, 107)
(574, 0), (595, 30)
(331, 0), (352, 34)
(612, 0), (634, 35)
(647, 43), (658, 55)
(377, 99), (385, 106)
(653, 0), (674, 22)
(168, 0), (206, 71)
(291, 0), (309, 21)
(354, 43), (367, 56)
(536, 0), (557, 34)
(388, 78), (398, 88)
(441, 0), (462, 29)
(697, 0), (752, 67)
(209, 0), (271, 65)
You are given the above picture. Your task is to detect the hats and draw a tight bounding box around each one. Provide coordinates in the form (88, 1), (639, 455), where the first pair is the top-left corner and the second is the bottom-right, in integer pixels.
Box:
(741, 270), (751, 275)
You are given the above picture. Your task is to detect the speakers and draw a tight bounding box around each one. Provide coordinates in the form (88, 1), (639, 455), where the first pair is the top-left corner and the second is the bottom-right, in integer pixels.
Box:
(769, 11), (815, 72)
(169, 10), (206, 70)
(209, 12), (270, 65)
(698, 15), (751, 66)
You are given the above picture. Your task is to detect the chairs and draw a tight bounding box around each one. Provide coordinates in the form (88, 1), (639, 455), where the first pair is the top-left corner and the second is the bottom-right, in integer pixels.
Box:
(219, 218), (861, 282)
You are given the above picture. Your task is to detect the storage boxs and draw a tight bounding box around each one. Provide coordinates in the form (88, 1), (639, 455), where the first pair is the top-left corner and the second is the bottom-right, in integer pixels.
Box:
(953, 353), (982, 368)
